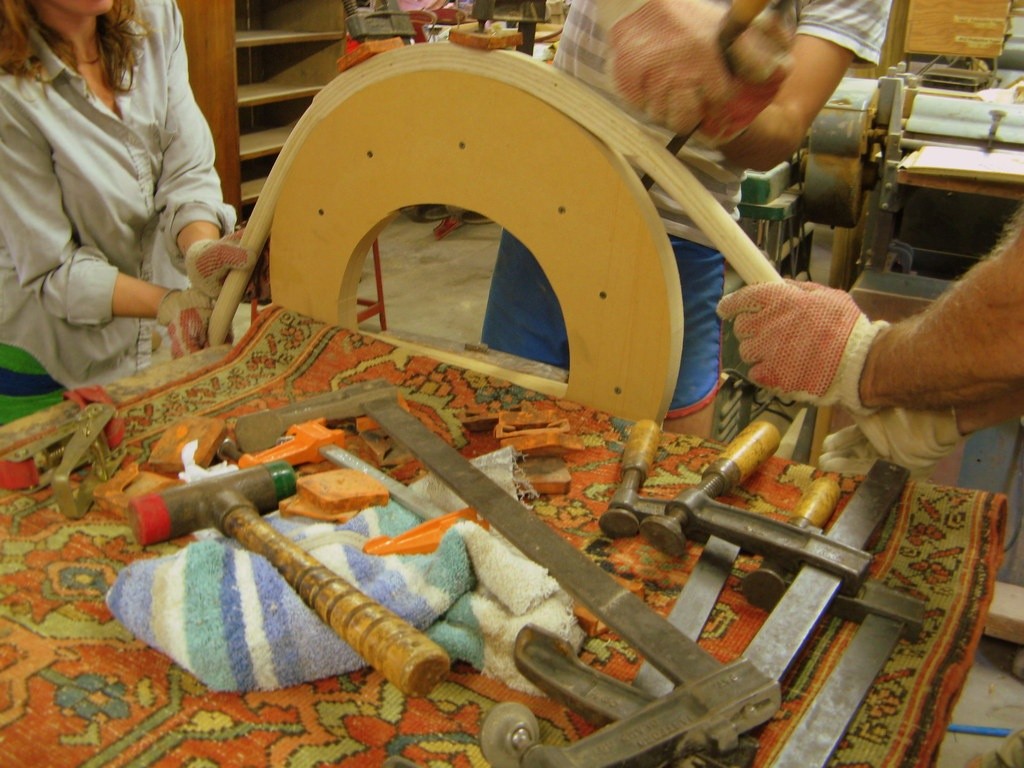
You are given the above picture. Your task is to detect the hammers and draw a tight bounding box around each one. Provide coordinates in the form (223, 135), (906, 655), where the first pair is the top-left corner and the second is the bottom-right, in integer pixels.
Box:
(128, 458), (451, 701)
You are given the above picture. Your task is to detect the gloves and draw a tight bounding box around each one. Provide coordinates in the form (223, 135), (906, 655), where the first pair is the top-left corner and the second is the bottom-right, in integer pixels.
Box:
(184, 229), (270, 303)
(602, 0), (794, 148)
(816, 399), (966, 475)
(715, 279), (887, 416)
(155, 289), (235, 359)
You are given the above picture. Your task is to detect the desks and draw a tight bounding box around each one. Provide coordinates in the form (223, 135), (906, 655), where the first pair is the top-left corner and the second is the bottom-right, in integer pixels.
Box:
(0, 324), (1010, 768)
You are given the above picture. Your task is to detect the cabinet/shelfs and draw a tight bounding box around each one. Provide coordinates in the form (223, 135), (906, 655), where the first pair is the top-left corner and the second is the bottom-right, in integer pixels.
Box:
(174, 0), (352, 303)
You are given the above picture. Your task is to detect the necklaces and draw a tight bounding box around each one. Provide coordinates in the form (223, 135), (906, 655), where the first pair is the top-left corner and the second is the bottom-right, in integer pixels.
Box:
(76, 41), (101, 67)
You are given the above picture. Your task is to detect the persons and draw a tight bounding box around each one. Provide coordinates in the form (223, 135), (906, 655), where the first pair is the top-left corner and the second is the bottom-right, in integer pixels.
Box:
(717, 210), (1024, 475)
(0, 0), (272, 428)
(480, 0), (893, 438)
